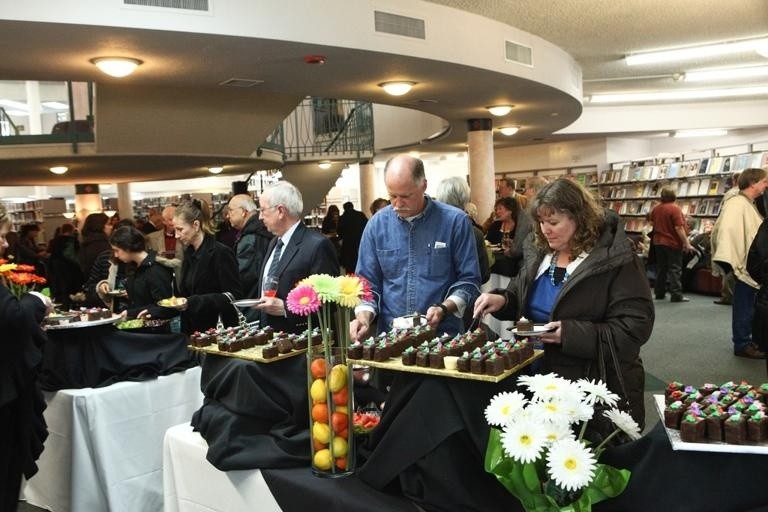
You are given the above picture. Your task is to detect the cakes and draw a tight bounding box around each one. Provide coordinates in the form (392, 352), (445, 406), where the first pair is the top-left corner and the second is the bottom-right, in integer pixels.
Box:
(664, 378), (768, 446)
(189, 322), (274, 354)
(68, 304), (113, 322)
(262, 327), (333, 360)
(347, 314), (542, 377)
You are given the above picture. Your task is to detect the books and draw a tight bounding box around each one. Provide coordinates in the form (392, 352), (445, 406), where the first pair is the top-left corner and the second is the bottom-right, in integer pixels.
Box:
(565, 150), (768, 233)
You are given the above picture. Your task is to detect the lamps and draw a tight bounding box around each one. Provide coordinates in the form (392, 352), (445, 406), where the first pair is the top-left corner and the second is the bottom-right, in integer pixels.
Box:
(683, 64), (767, 81)
(49, 164), (69, 176)
(375, 79), (418, 97)
(485, 103), (515, 117)
(87, 54), (143, 78)
(581, 83), (767, 104)
(623, 33), (767, 66)
(500, 125), (518, 136)
(317, 160), (333, 169)
(207, 164), (224, 174)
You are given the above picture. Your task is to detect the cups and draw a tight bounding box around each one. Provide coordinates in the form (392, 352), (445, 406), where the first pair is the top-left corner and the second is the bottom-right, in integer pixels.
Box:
(262, 275), (279, 299)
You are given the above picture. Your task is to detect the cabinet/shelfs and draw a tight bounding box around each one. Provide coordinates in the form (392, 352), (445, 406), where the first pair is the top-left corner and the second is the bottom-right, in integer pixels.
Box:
(5, 197), (68, 245)
(597, 152), (768, 258)
(492, 171), (597, 195)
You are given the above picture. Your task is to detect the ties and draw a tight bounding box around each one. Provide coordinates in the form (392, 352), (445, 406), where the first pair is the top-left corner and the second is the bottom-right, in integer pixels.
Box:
(267, 239), (283, 277)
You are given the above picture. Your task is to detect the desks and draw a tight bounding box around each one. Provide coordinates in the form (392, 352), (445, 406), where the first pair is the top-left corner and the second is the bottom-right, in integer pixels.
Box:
(339, 339), (545, 506)
(185, 337), (332, 469)
(40, 313), (124, 383)
(651, 392), (766, 512)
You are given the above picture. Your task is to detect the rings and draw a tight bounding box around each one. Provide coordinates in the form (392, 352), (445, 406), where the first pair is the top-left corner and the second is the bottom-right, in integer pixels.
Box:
(261, 304), (266, 311)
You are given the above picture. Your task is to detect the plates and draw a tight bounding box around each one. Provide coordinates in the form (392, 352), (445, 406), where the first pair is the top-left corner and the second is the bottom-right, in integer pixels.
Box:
(107, 290), (123, 295)
(511, 324), (553, 337)
(390, 314), (429, 328)
(157, 296), (188, 308)
(232, 298), (262, 308)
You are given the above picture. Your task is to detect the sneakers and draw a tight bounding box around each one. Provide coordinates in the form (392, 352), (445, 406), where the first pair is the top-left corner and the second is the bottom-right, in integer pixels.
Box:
(734, 342), (768, 359)
(714, 297), (731, 305)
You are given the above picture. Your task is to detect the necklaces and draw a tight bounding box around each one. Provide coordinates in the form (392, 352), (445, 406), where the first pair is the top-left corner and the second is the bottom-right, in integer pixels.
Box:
(545, 250), (572, 288)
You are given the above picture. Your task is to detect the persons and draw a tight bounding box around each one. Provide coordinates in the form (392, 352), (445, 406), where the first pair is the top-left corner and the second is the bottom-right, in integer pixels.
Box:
(0, 193), (276, 338)
(244, 179), (341, 336)
(625, 169), (768, 359)
(321, 174), (552, 284)
(345, 153), (481, 344)
(469, 176), (655, 448)
(1, 200), (53, 512)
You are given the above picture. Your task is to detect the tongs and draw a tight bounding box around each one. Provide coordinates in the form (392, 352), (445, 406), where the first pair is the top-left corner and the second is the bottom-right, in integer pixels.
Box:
(466, 303), (488, 334)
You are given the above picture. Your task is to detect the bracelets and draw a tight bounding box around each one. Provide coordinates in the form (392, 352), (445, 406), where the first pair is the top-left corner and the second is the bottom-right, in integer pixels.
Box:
(434, 303), (450, 316)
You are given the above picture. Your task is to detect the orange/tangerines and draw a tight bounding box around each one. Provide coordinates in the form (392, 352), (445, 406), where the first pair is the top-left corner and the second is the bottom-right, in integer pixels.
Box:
(313, 440), (327, 452)
(312, 404), (328, 423)
(337, 428), (349, 438)
(311, 358), (328, 378)
(335, 458), (347, 471)
(331, 387), (349, 406)
(330, 412), (349, 433)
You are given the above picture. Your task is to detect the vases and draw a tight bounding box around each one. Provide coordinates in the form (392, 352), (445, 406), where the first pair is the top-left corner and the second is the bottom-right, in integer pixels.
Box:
(306, 346), (357, 480)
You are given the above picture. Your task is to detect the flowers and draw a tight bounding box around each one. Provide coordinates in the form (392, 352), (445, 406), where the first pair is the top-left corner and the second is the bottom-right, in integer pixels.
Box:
(286, 271), (375, 474)
(0, 254), (50, 301)
(483, 371), (642, 512)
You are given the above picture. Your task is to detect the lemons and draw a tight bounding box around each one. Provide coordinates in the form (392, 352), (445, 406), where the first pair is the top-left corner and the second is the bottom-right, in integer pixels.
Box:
(311, 379), (327, 402)
(313, 421), (330, 444)
(313, 449), (331, 471)
(332, 436), (348, 457)
(329, 365), (346, 393)
(335, 405), (349, 414)
